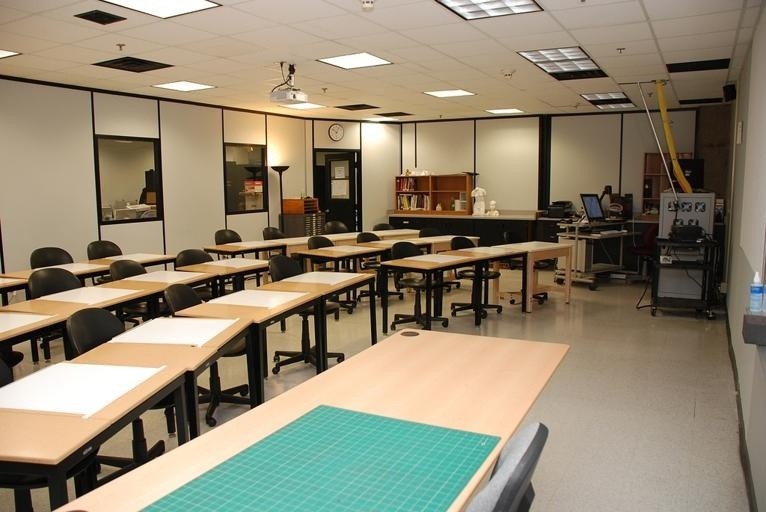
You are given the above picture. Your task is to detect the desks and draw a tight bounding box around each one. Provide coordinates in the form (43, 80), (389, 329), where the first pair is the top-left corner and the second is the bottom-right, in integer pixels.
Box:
(56, 329), (570, 510)
(168, 224), (384, 427)
(1, 240), (269, 511)
(357, 227), (575, 329)
(555, 221), (643, 291)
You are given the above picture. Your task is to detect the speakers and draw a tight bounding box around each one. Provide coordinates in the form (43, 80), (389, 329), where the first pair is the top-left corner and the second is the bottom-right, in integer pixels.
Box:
(722, 83), (737, 102)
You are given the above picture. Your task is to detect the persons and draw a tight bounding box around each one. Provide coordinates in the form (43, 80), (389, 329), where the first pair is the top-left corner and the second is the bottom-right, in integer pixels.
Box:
(471, 187), (487, 216)
(486, 200), (499, 216)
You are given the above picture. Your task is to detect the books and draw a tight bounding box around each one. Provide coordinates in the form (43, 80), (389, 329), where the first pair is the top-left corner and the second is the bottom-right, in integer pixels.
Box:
(397, 177), (429, 211)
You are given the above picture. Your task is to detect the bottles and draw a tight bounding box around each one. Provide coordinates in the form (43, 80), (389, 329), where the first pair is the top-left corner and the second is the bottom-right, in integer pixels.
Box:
(436, 203), (442, 211)
(750, 272), (764, 314)
(449, 200), (455, 211)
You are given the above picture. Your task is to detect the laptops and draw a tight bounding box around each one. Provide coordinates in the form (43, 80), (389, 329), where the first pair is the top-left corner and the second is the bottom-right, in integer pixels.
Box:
(579, 193), (624, 225)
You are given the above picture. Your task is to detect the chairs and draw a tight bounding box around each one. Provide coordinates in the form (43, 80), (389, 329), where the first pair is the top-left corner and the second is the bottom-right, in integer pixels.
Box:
(466, 422), (550, 510)
(101, 198), (156, 220)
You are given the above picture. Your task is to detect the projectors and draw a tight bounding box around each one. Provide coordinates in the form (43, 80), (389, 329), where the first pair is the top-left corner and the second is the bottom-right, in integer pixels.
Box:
(268, 90), (307, 105)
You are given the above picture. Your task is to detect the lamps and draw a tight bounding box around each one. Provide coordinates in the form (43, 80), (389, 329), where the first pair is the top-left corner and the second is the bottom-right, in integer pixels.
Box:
(244, 166), (263, 179)
(272, 166), (290, 214)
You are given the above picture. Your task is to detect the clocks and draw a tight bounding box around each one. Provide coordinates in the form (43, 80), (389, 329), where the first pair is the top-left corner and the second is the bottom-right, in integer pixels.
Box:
(328, 124), (345, 141)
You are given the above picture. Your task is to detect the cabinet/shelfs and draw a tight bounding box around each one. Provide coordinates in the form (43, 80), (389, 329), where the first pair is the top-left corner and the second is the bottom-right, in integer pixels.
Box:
(394, 173), (474, 216)
(650, 236), (720, 320)
